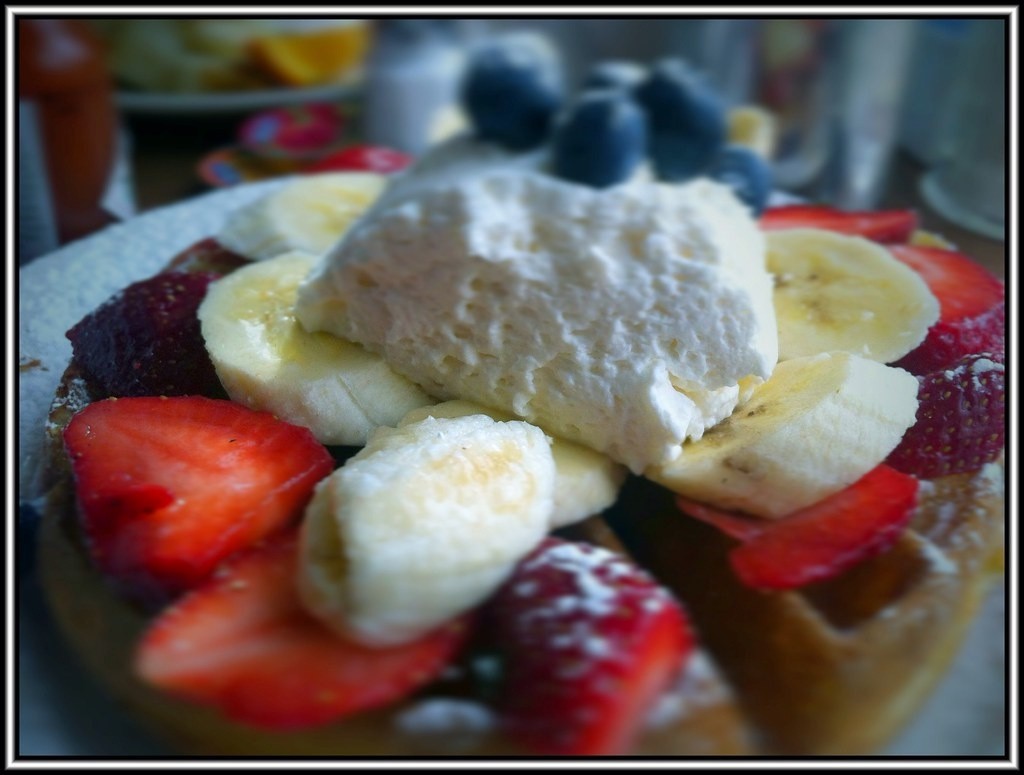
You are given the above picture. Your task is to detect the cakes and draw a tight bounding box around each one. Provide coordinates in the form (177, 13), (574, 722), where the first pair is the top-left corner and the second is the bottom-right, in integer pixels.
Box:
(38, 166), (1006, 762)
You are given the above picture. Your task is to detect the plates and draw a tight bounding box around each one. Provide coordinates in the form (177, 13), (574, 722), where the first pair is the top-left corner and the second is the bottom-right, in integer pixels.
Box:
(18, 173), (1009, 753)
